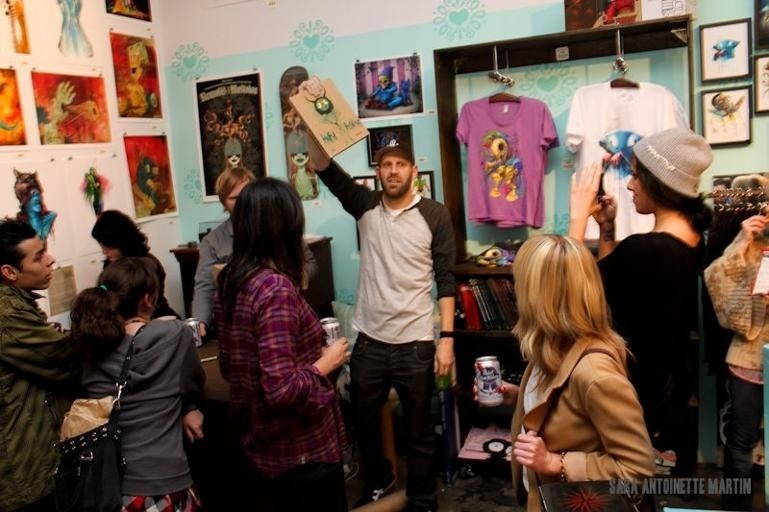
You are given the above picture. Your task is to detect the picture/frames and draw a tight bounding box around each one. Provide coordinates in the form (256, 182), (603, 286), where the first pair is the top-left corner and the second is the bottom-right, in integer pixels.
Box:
(352, 124), (436, 252)
(698, 1), (769, 149)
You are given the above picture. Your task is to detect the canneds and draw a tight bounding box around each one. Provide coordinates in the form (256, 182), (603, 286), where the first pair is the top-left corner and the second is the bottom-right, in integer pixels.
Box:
(475, 355), (504, 407)
(319, 318), (342, 346)
(184, 318), (203, 350)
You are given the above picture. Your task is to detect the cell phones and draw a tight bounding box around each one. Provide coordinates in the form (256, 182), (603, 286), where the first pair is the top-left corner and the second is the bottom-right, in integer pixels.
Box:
(598, 172), (607, 197)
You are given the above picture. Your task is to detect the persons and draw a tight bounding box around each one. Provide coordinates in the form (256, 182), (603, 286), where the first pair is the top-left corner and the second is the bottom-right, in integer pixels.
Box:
(91, 210), (182, 320)
(298, 77), (459, 511)
(473, 233), (656, 512)
(703, 206), (768, 512)
(0, 218), (77, 512)
(70, 255), (205, 512)
(567, 124), (713, 512)
(212, 178), (352, 511)
(191, 166), (318, 338)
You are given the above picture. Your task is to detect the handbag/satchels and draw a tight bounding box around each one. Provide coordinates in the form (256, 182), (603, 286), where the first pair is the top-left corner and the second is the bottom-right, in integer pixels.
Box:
(60, 423), (126, 512)
(537, 480), (656, 512)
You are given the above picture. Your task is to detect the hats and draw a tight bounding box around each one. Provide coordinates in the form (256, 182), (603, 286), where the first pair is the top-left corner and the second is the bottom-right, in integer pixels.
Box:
(373, 139), (414, 165)
(633, 127), (712, 198)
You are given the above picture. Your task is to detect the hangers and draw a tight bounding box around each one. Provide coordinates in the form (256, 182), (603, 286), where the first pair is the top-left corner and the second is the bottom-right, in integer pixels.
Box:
(607, 58), (637, 90)
(485, 69), (519, 104)
(712, 186), (769, 213)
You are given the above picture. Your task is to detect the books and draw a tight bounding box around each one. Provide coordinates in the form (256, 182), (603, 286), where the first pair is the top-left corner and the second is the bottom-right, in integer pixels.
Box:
(287, 78), (371, 162)
(459, 277), (518, 331)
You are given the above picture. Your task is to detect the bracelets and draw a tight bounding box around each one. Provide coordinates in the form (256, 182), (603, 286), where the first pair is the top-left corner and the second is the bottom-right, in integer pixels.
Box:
(439, 331), (454, 338)
(560, 451), (567, 478)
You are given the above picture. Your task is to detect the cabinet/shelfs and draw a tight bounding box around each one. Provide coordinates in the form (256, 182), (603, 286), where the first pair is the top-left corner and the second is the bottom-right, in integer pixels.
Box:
(450, 264), (698, 479)
(170, 236), (336, 324)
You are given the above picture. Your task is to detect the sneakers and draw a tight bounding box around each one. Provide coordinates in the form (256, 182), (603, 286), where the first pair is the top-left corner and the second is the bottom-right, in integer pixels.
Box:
(342, 463), (438, 511)
